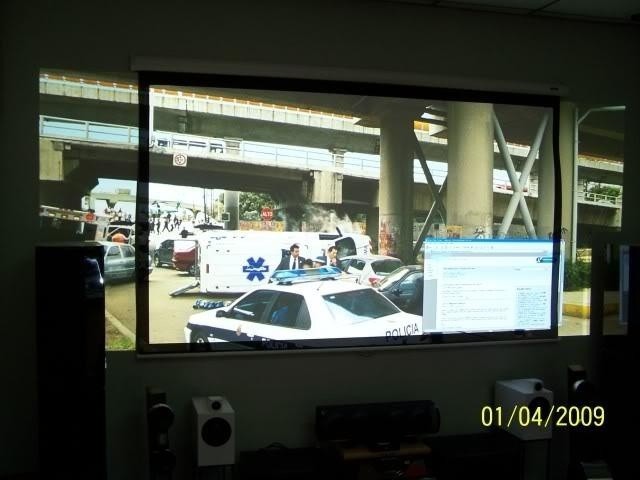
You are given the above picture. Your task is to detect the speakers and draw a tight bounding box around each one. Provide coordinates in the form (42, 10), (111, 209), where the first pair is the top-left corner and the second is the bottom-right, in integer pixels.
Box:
(568, 364), (597, 480)
(191, 394), (236, 467)
(146, 390), (176, 480)
(315, 400), (438, 449)
(494, 377), (553, 440)
(33, 239), (108, 480)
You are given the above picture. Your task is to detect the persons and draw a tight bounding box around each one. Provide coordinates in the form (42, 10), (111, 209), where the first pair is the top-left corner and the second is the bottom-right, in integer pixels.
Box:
(85, 208), (96, 222)
(276, 245), (343, 270)
(103, 207), (183, 236)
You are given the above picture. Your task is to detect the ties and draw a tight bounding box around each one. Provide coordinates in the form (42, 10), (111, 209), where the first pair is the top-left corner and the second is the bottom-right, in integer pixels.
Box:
(292, 258), (296, 269)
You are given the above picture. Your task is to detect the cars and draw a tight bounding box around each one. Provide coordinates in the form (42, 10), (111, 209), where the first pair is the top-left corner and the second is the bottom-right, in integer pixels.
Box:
(154, 239), (199, 275)
(184, 254), (424, 344)
(87, 240), (152, 283)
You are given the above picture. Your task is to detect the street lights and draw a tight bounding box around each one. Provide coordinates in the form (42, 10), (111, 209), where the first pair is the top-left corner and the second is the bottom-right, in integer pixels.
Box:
(571, 105), (625, 262)
